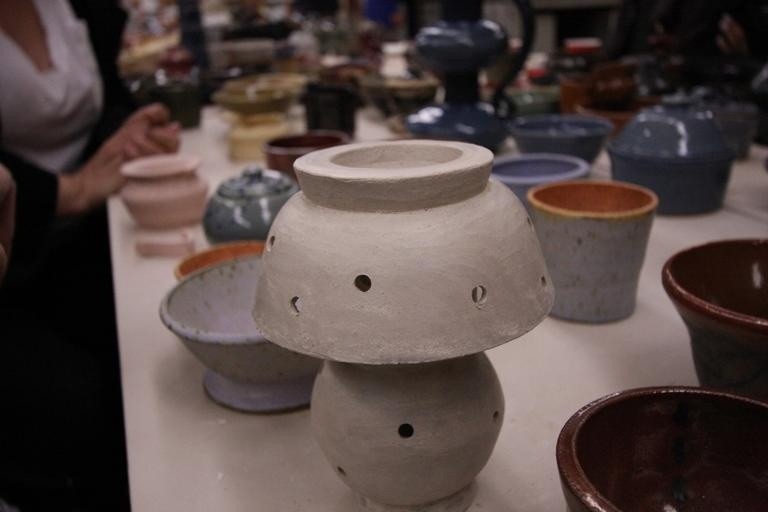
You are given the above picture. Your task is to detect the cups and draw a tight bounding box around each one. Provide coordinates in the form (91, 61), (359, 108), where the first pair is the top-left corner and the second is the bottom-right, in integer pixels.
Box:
(261, 128), (351, 181)
(488, 113), (660, 324)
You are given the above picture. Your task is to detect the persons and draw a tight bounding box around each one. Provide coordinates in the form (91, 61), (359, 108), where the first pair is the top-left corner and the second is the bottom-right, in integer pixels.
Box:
(1, 1), (103, 180)
(4, 104), (180, 222)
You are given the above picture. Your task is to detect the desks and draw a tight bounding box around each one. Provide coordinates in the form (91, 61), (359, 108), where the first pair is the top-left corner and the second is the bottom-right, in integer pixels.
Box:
(106, 122), (768, 512)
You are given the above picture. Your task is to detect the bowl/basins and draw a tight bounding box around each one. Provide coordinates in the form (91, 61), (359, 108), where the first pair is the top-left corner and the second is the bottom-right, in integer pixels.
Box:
(159, 255), (315, 414)
(661, 237), (768, 392)
(557, 383), (767, 512)
